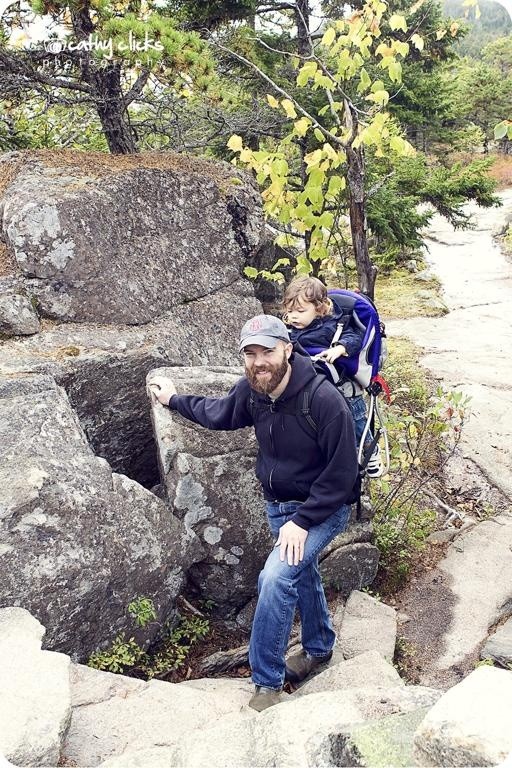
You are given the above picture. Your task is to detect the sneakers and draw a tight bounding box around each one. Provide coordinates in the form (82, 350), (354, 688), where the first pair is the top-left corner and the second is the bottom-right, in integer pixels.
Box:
(365, 441), (384, 479)
(248, 684), (282, 712)
(285, 647), (334, 683)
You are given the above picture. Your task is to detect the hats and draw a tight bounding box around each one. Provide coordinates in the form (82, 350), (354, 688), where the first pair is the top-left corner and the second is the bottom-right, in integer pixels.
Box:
(238, 315), (292, 355)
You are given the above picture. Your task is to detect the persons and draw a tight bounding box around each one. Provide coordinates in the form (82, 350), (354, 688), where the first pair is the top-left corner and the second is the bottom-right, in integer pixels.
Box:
(274, 275), (386, 480)
(144, 314), (364, 714)
